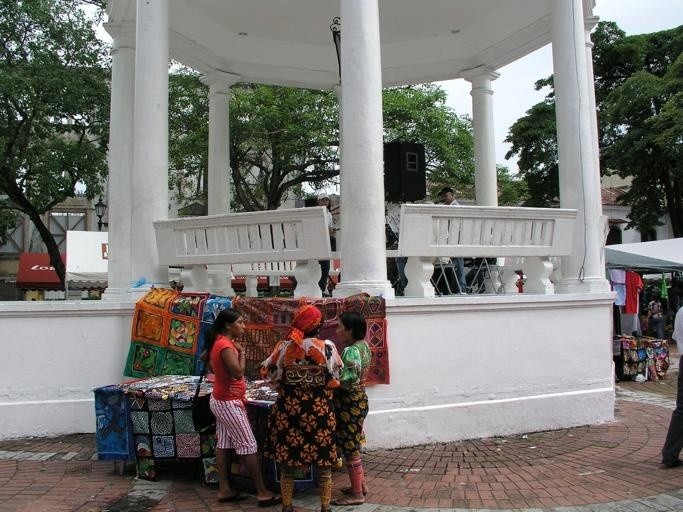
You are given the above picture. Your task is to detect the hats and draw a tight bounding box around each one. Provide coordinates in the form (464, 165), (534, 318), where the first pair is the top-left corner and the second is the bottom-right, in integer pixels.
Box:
(437, 188), (453, 196)
(318, 193), (330, 200)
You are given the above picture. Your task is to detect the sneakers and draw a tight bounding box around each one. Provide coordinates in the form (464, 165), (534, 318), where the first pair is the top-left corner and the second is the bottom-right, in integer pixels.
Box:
(662, 458), (682, 466)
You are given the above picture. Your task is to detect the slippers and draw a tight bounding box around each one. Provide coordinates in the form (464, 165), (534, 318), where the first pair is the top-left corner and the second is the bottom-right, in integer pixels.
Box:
(258, 493), (282, 507)
(341, 487), (367, 495)
(218, 491), (246, 503)
(330, 498), (362, 506)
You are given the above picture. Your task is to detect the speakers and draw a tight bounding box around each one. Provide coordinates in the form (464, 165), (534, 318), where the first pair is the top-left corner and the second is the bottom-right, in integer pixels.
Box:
(383, 142), (427, 203)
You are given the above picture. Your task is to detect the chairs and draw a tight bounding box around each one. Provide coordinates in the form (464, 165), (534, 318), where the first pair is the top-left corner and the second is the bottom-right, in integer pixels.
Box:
(385, 214), (508, 296)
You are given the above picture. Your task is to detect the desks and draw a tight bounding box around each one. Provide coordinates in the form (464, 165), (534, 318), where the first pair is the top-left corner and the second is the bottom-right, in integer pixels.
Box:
(613, 335), (669, 383)
(91, 377), (316, 498)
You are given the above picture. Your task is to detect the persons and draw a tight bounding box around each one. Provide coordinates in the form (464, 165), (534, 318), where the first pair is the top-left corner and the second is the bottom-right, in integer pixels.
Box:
(640, 291), (683, 467)
(199, 310), (283, 512)
(257, 304), (343, 512)
(304, 187), (525, 296)
(332, 310), (372, 506)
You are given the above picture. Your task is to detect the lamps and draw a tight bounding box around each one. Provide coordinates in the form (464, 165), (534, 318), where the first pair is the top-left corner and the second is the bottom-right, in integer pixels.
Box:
(94, 192), (108, 231)
(329, 16), (341, 105)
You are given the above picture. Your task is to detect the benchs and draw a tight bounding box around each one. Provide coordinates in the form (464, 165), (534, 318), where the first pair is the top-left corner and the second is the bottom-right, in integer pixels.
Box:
(151, 205), (342, 299)
(386, 204), (579, 296)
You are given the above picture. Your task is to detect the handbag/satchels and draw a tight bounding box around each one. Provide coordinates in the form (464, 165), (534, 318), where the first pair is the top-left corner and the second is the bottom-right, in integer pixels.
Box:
(192, 393), (215, 430)
